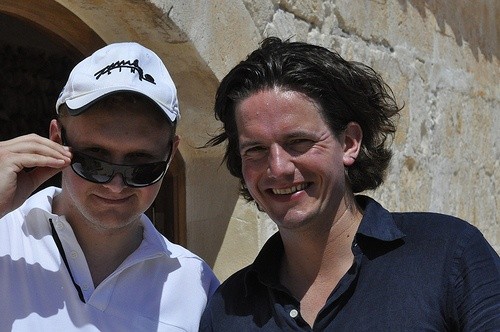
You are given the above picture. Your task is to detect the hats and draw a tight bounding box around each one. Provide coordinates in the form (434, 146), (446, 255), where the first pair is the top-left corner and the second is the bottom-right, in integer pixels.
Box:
(56, 41), (182, 123)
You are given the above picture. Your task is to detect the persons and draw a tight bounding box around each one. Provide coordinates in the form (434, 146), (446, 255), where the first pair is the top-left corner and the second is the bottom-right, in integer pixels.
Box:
(0, 42), (221, 332)
(189, 36), (500, 332)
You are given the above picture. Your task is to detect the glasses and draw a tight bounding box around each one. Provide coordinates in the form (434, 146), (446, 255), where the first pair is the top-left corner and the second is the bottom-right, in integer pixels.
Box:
(61, 125), (173, 189)
